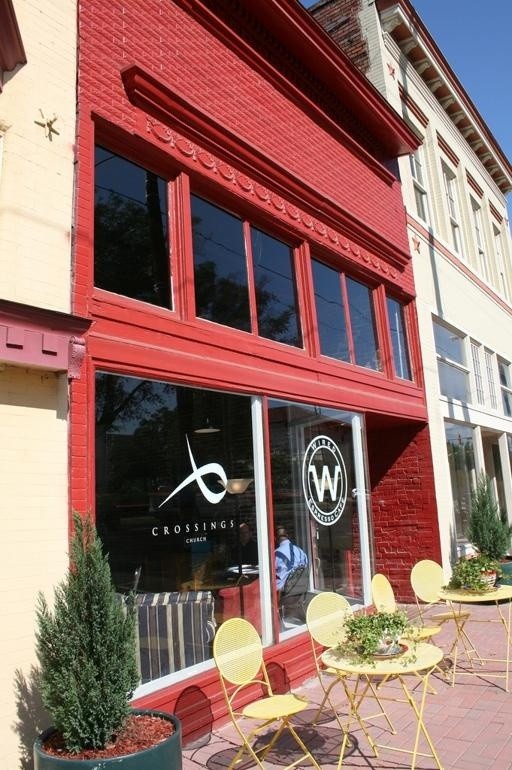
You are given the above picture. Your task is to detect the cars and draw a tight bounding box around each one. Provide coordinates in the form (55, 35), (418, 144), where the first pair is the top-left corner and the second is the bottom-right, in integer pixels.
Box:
(132, 462), (370, 513)
(452, 494), (478, 525)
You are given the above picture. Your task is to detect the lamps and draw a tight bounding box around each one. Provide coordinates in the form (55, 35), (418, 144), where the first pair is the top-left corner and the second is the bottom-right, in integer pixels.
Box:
(193, 390), (255, 495)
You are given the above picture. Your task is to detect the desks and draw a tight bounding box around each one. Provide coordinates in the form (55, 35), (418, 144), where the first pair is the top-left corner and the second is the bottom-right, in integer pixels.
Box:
(439, 583), (512, 693)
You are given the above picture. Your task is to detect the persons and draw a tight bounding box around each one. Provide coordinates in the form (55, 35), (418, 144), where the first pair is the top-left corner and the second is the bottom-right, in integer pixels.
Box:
(274, 526), (308, 607)
(225, 522), (258, 584)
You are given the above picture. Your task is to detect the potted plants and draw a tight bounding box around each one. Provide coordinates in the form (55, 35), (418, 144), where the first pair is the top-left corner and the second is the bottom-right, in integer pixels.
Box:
(463, 468), (512, 581)
(440, 552), (499, 593)
(30, 508), (184, 770)
